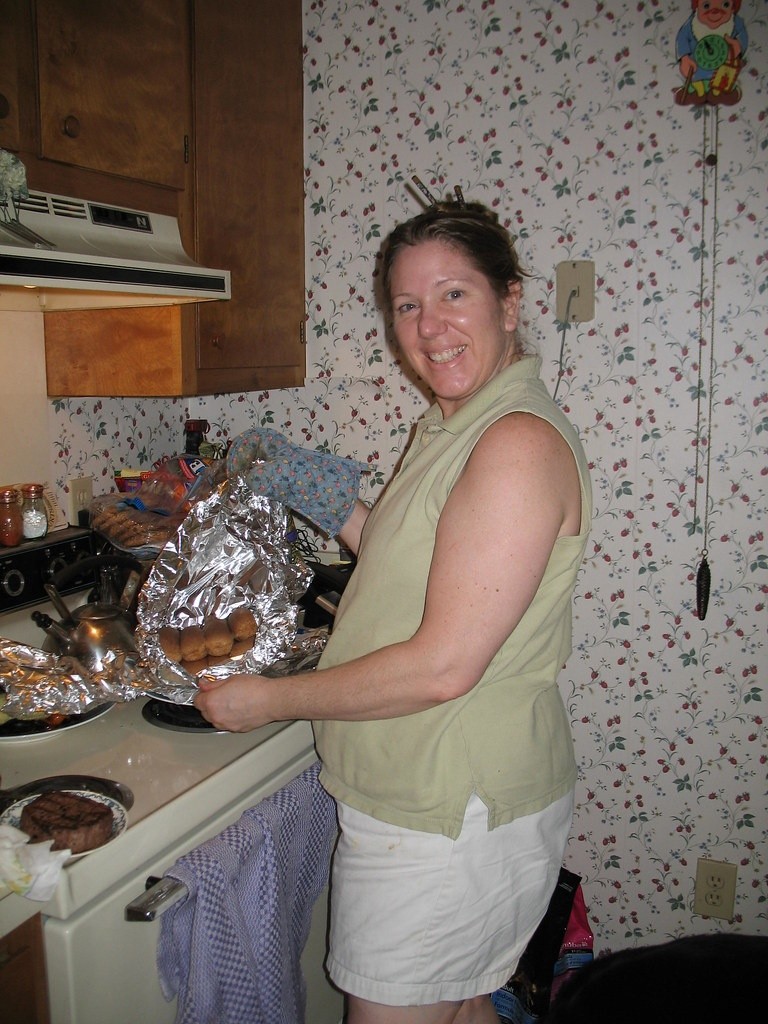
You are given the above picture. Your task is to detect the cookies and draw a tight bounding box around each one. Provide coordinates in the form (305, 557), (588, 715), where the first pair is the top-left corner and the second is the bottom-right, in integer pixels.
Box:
(90, 495), (196, 546)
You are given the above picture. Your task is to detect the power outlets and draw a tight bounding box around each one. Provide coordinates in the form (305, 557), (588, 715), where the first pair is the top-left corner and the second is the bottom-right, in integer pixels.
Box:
(556, 259), (596, 324)
(693, 858), (737, 920)
(70, 476), (93, 524)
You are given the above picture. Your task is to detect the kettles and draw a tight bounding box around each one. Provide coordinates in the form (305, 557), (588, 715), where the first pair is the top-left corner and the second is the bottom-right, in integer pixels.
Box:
(29, 552), (146, 670)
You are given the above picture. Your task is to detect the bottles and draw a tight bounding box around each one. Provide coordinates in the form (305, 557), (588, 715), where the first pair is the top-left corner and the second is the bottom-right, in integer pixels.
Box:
(0, 486), (22, 548)
(21, 483), (49, 541)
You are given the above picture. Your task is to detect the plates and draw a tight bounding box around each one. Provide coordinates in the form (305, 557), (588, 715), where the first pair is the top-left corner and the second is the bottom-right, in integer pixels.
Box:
(142, 698), (233, 734)
(0, 790), (129, 864)
(1, 774), (134, 812)
(0, 693), (116, 743)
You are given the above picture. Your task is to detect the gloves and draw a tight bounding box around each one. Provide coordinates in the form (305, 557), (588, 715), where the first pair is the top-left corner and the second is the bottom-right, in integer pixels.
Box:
(225, 426), (369, 538)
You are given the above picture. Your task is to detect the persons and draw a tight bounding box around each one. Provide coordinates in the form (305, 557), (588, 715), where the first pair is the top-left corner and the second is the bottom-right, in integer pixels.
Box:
(196, 175), (591, 1024)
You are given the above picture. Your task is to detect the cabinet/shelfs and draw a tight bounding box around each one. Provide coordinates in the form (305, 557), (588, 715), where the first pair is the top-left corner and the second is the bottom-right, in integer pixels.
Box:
(0, 0), (310, 397)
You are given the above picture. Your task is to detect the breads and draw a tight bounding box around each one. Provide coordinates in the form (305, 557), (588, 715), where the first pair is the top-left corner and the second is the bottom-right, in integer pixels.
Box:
(20, 789), (112, 852)
(156, 608), (258, 675)
